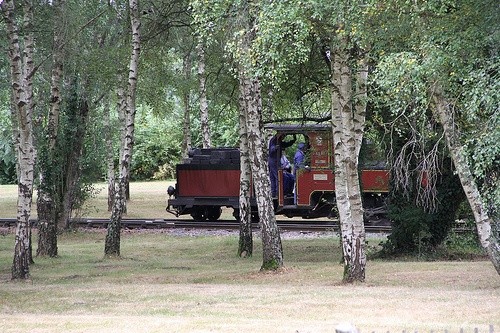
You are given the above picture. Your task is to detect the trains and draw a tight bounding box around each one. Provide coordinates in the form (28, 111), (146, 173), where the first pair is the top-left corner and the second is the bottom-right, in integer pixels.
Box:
(167, 123), (436, 228)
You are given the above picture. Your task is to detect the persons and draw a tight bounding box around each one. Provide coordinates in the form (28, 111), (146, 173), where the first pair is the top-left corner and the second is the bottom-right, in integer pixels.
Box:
(293, 142), (307, 184)
(280, 147), (295, 199)
(267, 132), (297, 202)
